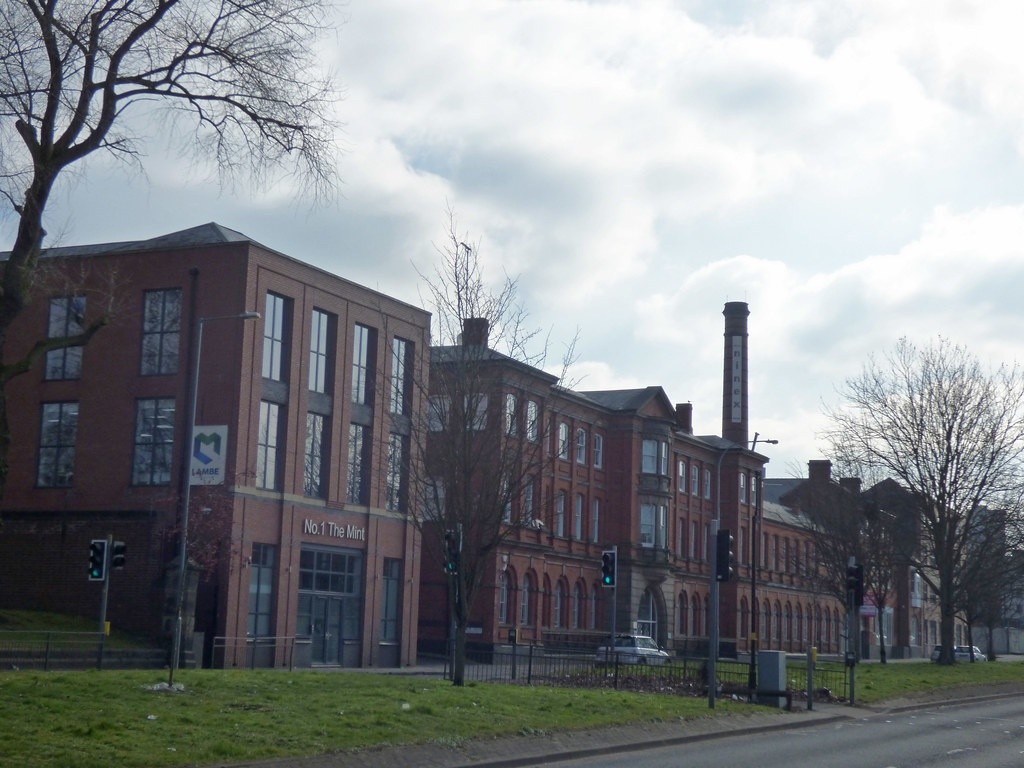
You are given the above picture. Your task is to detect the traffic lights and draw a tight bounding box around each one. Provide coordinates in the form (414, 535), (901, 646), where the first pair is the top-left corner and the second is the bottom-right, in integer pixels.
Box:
(718, 528), (733, 581)
(601, 550), (616, 588)
(85, 540), (107, 581)
(444, 529), (463, 575)
(111, 540), (126, 575)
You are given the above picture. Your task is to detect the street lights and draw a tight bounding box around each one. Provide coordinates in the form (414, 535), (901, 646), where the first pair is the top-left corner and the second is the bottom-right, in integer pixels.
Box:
(715, 440), (778, 663)
(169, 312), (262, 667)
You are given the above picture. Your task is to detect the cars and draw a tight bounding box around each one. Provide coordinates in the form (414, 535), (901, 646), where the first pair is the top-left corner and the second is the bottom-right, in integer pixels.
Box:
(954, 646), (986, 663)
(930, 644), (956, 660)
(597, 635), (672, 666)
